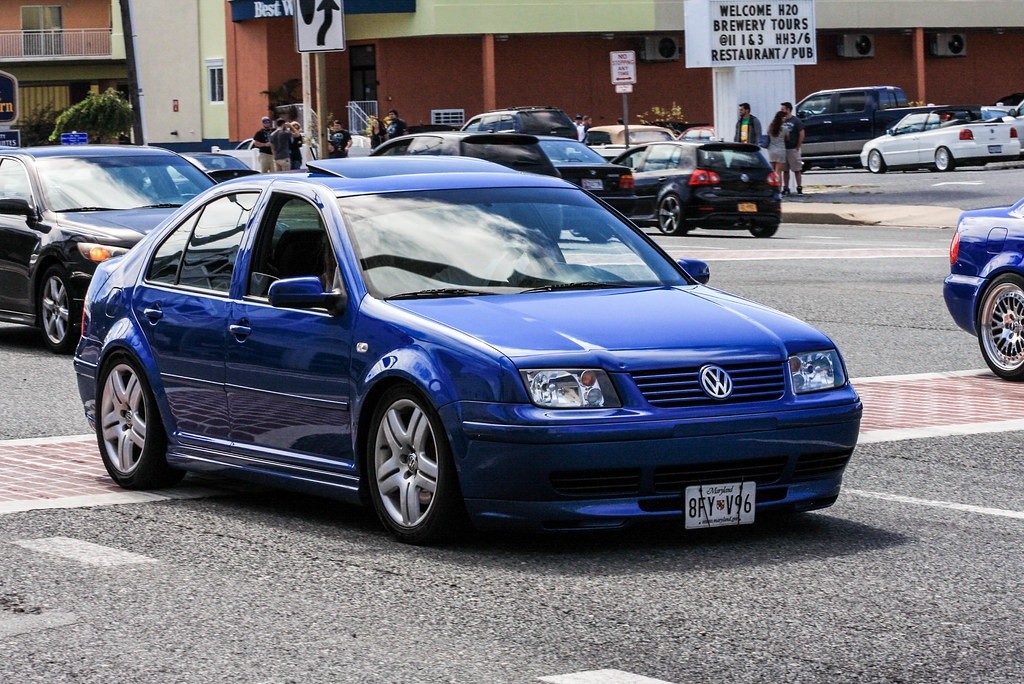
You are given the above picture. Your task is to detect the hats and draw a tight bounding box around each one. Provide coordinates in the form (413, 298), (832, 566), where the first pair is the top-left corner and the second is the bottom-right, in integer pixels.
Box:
(261, 116), (270, 121)
(576, 113), (582, 119)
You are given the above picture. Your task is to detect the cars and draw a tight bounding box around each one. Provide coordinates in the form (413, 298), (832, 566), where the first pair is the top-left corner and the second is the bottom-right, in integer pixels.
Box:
(0, 145), (291, 354)
(174, 151), (261, 201)
(993, 93), (1024, 149)
(861, 106), (1020, 172)
(582, 125), (716, 146)
(535, 135), (638, 244)
(943, 197), (1024, 381)
(74, 155), (863, 547)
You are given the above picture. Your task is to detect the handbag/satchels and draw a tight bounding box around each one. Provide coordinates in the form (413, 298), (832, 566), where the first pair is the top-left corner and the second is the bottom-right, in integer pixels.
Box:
(758, 134), (770, 147)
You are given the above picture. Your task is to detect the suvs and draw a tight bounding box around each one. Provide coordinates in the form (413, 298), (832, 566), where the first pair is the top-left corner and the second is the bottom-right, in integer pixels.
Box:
(370, 131), (561, 243)
(606, 140), (783, 237)
(457, 106), (579, 138)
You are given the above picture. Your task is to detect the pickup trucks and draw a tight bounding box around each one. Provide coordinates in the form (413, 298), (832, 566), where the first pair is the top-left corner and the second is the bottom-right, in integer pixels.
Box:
(767, 84), (985, 173)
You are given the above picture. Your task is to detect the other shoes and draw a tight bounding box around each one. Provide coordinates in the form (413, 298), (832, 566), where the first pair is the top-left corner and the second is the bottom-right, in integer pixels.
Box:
(796, 185), (803, 195)
(782, 185), (790, 194)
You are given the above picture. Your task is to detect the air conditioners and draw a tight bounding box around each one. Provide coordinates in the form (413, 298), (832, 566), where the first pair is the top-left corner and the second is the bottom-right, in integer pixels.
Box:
(836, 33), (874, 58)
(929, 32), (968, 57)
(639, 35), (680, 63)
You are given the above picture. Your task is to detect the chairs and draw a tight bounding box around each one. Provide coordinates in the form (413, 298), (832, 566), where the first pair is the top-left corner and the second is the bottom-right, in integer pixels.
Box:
(273, 228), (329, 281)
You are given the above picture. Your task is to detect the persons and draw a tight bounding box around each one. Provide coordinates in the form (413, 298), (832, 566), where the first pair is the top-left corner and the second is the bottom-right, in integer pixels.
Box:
(252, 110), (410, 173)
(766, 111), (790, 200)
(734, 103), (762, 146)
(780, 102), (805, 196)
(573, 114), (625, 142)
